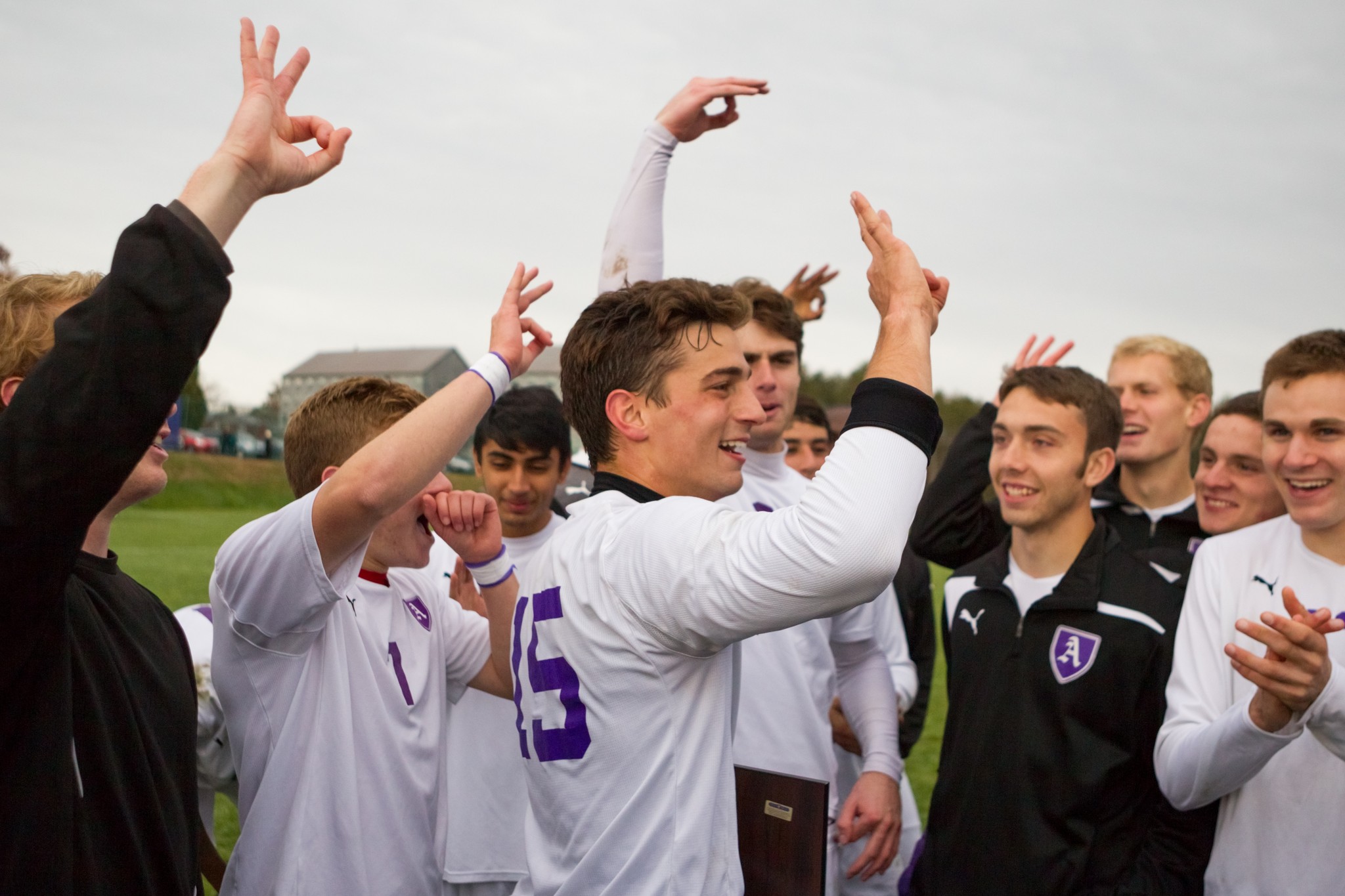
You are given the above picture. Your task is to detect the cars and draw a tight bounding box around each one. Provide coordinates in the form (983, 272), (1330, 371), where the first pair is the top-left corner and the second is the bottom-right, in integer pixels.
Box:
(180, 426), (285, 457)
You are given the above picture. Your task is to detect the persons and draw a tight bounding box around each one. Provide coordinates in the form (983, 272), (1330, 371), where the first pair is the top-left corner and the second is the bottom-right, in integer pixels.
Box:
(760, 256), (932, 895)
(404, 384), (593, 896)
(1161, 390), (1303, 608)
(494, 190), (962, 895)
(0, 13), (355, 894)
(582, 76), (907, 895)
(1159, 333), (1344, 895)
(144, 600), (248, 896)
(899, 363), (1219, 896)
(910, 322), (1232, 630)
(207, 263), (554, 896)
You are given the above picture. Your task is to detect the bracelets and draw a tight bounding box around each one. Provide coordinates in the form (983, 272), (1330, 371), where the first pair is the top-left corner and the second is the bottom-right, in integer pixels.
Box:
(460, 348), (513, 404)
(463, 539), (514, 589)
